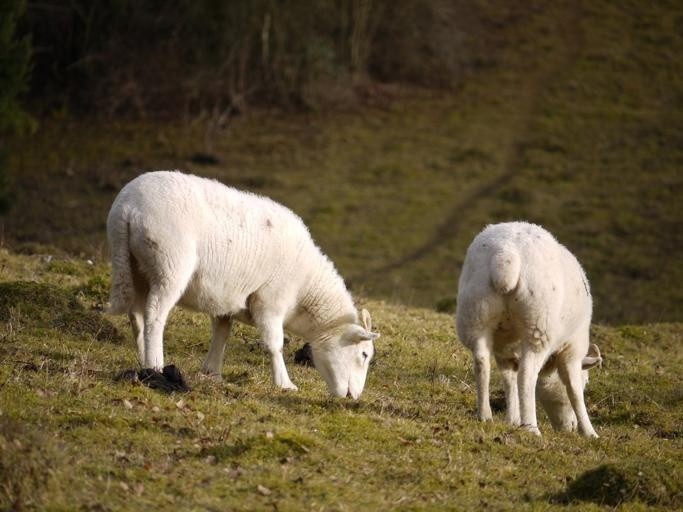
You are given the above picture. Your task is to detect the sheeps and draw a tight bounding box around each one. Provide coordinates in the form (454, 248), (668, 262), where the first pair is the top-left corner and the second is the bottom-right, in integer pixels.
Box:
(454, 220), (603, 441)
(105, 169), (382, 404)
